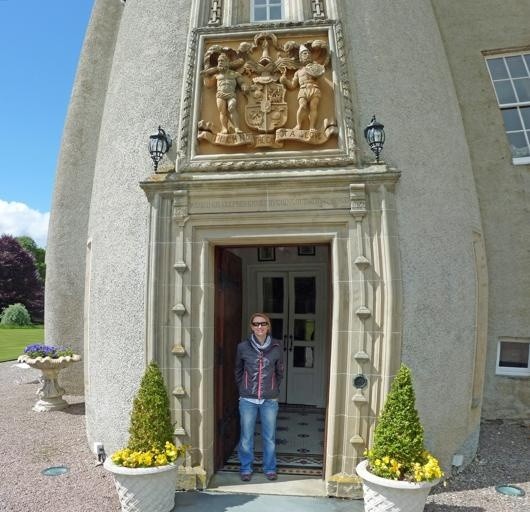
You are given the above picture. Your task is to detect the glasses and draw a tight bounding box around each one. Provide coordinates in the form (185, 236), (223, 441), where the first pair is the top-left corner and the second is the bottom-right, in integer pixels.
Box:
(251, 320), (269, 327)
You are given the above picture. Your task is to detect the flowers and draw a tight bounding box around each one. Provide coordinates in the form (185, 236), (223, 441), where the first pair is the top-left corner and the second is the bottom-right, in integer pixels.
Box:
(362, 362), (445, 484)
(111, 359), (183, 467)
(24, 340), (73, 358)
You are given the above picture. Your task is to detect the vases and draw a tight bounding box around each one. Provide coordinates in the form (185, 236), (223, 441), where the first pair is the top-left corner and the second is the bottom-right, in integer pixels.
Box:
(355, 458), (444, 512)
(103, 447), (186, 512)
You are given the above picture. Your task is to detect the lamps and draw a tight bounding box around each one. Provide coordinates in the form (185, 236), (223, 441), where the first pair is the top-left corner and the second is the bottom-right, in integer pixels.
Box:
(147, 126), (172, 171)
(363, 114), (386, 164)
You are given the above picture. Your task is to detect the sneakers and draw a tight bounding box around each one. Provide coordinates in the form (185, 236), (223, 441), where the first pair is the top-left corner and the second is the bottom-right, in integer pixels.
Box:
(265, 472), (278, 480)
(240, 472), (252, 482)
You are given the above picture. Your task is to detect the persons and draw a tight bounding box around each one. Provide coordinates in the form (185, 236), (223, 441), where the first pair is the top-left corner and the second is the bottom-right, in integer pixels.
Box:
(279, 45), (326, 133)
(233, 313), (287, 482)
(200, 53), (249, 135)
